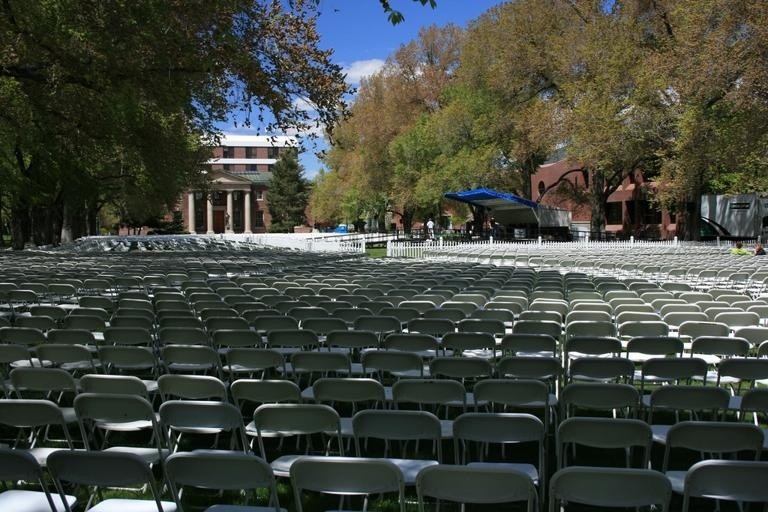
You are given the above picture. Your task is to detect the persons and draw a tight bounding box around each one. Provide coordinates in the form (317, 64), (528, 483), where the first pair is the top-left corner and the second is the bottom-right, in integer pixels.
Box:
(753, 242), (766, 256)
(426, 218), (435, 240)
(729, 241), (752, 255)
(488, 215), (496, 240)
